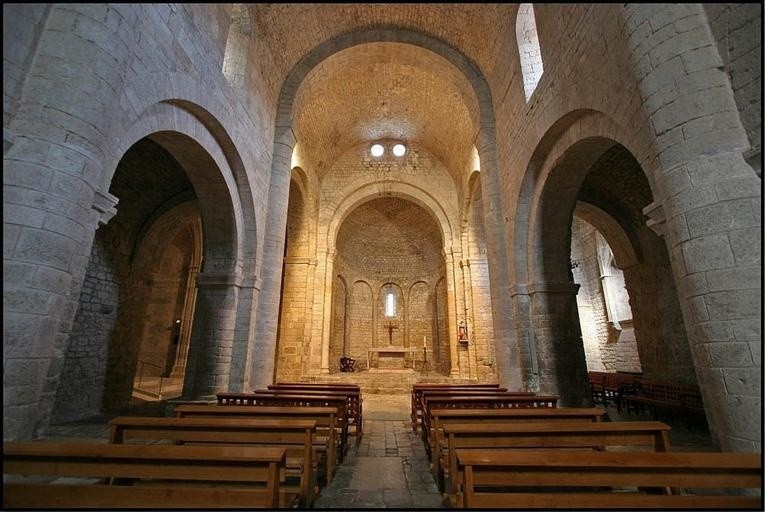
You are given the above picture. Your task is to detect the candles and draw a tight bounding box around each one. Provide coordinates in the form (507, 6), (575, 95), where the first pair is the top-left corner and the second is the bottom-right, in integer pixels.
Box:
(424, 336), (426, 347)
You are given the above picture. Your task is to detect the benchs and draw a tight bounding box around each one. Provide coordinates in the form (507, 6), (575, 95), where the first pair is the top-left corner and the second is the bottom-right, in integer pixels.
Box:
(102, 417), (322, 508)
(173, 405), (338, 487)
(587, 371), (705, 417)
(439, 422), (683, 495)
(3, 442), (299, 508)
(455, 448), (761, 508)
(428, 409), (607, 488)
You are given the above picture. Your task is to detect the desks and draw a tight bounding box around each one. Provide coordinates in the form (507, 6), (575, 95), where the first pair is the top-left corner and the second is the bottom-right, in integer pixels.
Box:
(367, 348), (418, 369)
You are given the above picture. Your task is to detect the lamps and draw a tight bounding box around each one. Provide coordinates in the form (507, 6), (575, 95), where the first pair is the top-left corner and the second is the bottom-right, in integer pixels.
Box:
(384, 294), (399, 344)
